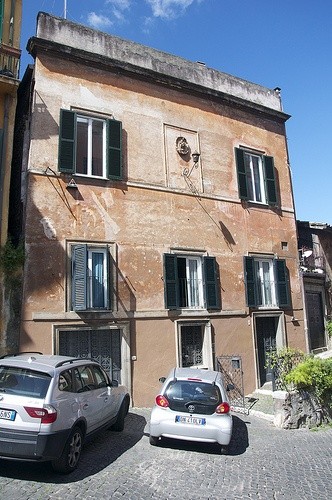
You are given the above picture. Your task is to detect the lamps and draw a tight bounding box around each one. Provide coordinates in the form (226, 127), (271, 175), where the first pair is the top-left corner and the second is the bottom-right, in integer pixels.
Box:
(183, 150), (200, 178)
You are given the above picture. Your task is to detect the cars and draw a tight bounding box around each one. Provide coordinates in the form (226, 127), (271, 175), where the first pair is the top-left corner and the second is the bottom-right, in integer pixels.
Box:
(147, 366), (236, 456)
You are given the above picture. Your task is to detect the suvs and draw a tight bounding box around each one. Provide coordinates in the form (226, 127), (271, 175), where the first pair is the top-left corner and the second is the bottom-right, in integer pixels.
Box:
(0, 350), (130, 477)
